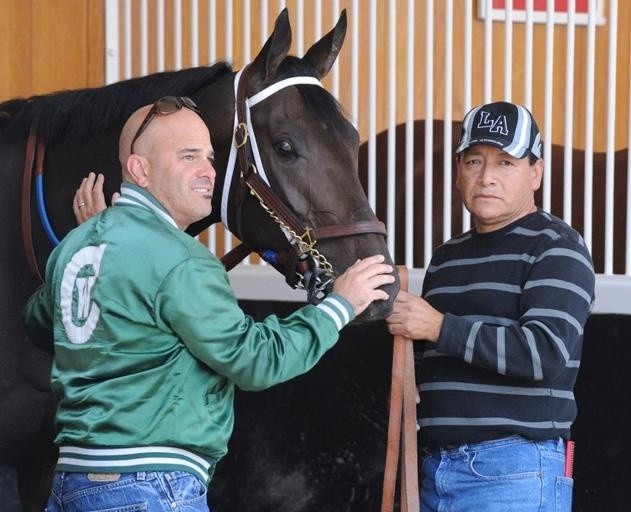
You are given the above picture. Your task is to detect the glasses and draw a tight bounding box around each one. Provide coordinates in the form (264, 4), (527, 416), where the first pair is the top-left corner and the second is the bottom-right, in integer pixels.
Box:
(130, 95), (200, 155)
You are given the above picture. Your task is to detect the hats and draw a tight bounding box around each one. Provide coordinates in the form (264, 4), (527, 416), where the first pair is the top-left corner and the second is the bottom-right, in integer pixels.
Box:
(455, 101), (541, 158)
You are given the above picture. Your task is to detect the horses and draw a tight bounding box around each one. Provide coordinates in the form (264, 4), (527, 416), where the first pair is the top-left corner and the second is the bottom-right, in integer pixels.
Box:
(1, 4), (403, 512)
(358, 117), (630, 271)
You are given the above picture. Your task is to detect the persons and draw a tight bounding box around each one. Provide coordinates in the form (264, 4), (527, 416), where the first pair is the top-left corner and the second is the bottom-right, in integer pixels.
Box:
(13, 93), (401, 512)
(383, 100), (600, 512)
(78, 202), (85, 208)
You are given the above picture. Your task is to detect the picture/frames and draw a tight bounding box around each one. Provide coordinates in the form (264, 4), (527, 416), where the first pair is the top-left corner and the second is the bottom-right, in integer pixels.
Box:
(476, 0), (606, 28)
